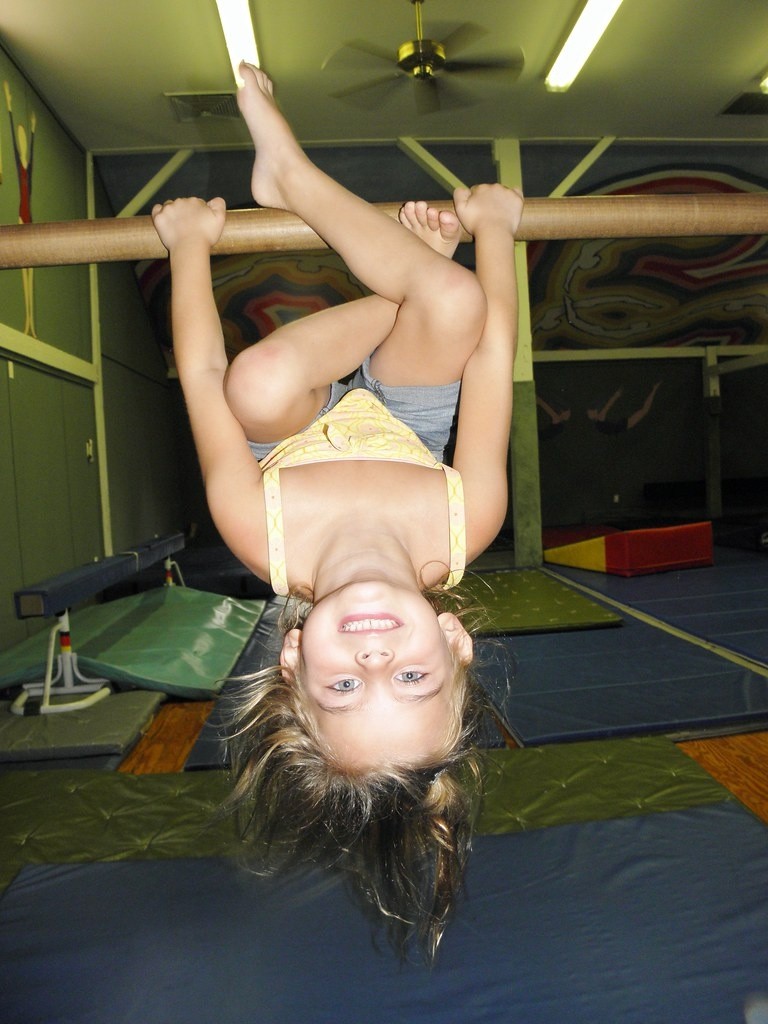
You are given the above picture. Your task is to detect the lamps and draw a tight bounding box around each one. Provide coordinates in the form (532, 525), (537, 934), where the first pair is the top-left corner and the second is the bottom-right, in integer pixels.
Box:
(215, 0), (261, 85)
(543, 0), (622, 93)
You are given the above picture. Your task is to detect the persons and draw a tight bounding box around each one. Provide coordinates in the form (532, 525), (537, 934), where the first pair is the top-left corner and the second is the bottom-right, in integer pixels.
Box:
(148, 61), (524, 970)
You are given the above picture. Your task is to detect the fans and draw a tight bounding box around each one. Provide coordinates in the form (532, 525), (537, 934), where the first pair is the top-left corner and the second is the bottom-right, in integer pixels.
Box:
(321, 0), (525, 115)
